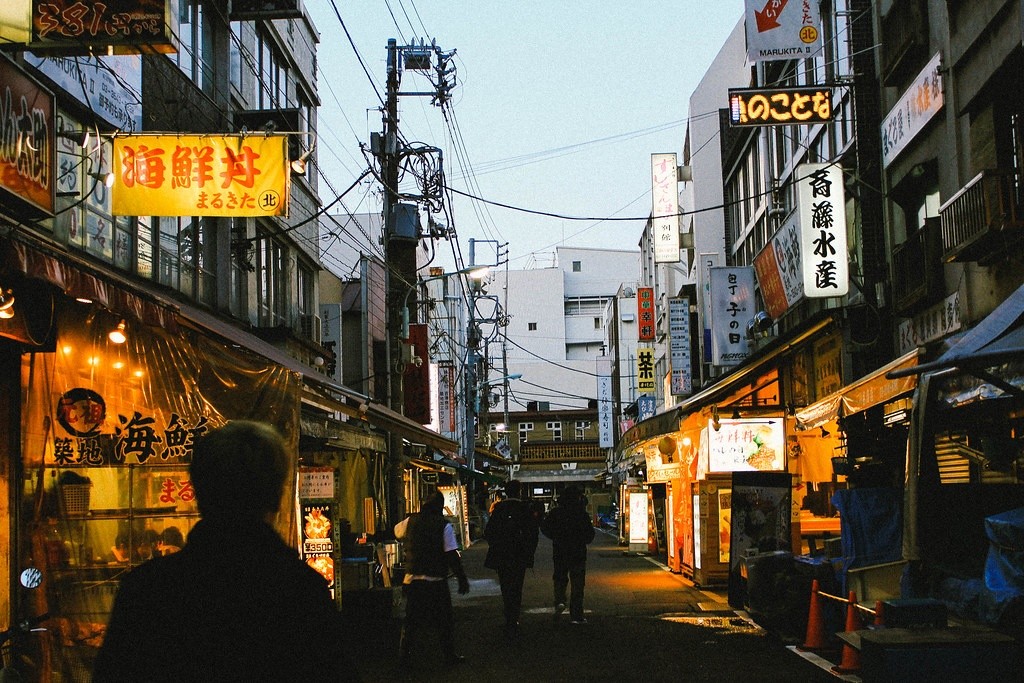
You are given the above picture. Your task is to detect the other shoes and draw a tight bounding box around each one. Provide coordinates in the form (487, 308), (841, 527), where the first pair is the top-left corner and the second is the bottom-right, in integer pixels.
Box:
(446, 655), (465, 666)
(571, 618), (587, 625)
(553, 603), (565, 620)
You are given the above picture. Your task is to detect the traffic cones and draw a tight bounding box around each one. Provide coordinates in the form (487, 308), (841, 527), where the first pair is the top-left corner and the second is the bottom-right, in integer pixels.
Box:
(797, 580), (833, 652)
(831, 590), (886, 675)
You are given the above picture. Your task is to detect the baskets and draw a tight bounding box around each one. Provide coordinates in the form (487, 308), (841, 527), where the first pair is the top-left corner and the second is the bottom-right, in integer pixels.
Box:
(56, 483), (89, 516)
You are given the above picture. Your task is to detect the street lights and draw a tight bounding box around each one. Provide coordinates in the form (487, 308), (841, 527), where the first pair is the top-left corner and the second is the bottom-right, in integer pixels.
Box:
(388, 264), (491, 536)
(466, 374), (523, 468)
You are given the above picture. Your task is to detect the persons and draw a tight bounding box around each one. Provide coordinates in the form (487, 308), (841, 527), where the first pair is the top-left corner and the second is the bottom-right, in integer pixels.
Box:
(541, 487), (595, 623)
(484, 480), (539, 631)
(90, 421), (338, 683)
(394, 490), (470, 665)
(105, 526), (184, 562)
